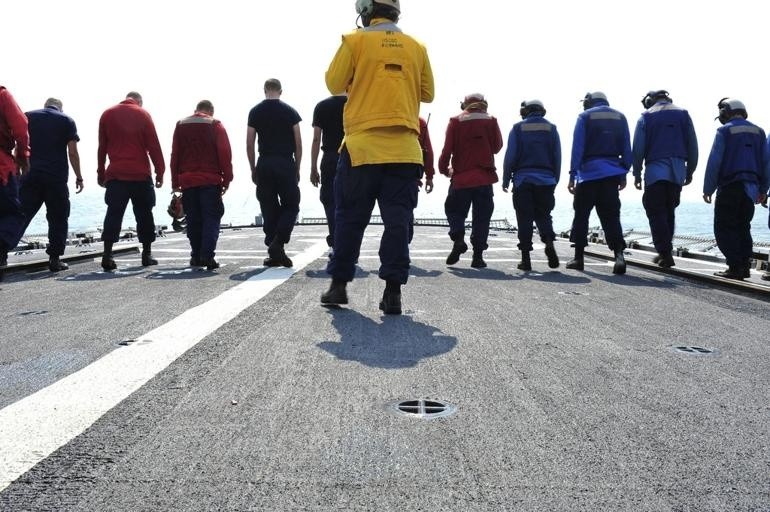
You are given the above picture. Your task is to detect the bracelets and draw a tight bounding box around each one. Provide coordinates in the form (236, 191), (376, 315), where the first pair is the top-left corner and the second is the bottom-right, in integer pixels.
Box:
(76, 179), (83, 182)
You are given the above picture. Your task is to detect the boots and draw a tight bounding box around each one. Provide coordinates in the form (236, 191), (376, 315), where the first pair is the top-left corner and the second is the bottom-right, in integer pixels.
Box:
(471, 248), (487, 267)
(49, 254), (70, 271)
(518, 249), (531, 271)
(565, 246), (584, 271)
(189, 253), (219, 268)
(446, 235), (467, 264)
(101, 240), (117, 269)
(545, 239), (559, 268)
(653, 250), (675, 266)
(264, 234), (293, 268)
(379, 282), (402, 315)
(715, 263), (750, 281)
(321, 269), (349, 305)
(612, 248), (626, 274)
(142, 241), (158, 266)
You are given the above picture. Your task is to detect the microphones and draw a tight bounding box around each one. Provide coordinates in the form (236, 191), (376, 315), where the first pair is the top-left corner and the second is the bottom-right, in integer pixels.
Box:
(355, 6), (368, 29)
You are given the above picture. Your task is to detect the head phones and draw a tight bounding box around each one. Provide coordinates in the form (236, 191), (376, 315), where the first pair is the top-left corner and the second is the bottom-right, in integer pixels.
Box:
(644, 95), (673, 108)
(460, 101), (488, 110)
(519, 106), (546, 117)
(355, 0), (376, 17)
(718, 98), (729, 124)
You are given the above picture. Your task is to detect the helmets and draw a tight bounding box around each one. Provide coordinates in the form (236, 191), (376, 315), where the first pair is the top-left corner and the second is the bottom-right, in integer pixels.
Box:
(460, 92), (489, 110)
(642, 88), (673, 109)
(169, 190), (186, 219)
(520, 99), (546, 120)
(580, 92), (608, 109)
(355, 0), (400, 28)
(715, 97), (747, 123)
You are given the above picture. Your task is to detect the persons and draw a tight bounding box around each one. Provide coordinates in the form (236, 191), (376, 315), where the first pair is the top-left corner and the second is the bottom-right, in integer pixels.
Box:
(703, 97), (766, 281)
(320, 0), (435, 314)
(439, 95), (502, 268)
(170, 99), (232, 270)
(97, 91), (166, 270)
(632, 89), (699, 267)
(565, 90), (633, 273)
(502, 99), (562, 271)
(246, 78), (302, 269)
(1, 97), (84, 272)
(310, 89), (349, 259)
(758, 132), (770, 281)
(0, 84), (31, 221)
(406, 118), (436, 269)
